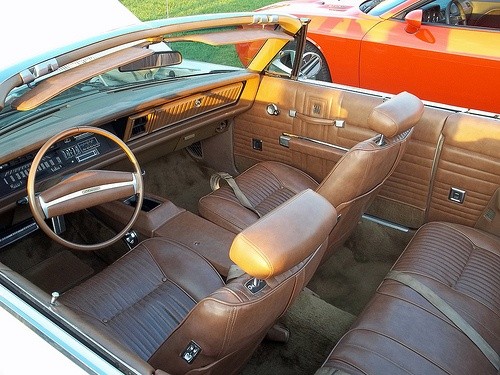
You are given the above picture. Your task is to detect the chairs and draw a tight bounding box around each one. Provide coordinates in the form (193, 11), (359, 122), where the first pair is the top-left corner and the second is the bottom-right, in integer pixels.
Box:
(53, 188), (336, 374)
(198, 91), (424, 268)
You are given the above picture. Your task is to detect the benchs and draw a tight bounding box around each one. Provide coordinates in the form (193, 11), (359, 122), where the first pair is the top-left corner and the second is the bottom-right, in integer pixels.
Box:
(315, 220), (499, 375)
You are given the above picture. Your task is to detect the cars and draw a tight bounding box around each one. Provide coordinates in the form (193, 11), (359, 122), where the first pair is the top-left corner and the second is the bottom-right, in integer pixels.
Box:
(235, 0), (500, 116)
(0, 0), (500, 375)
